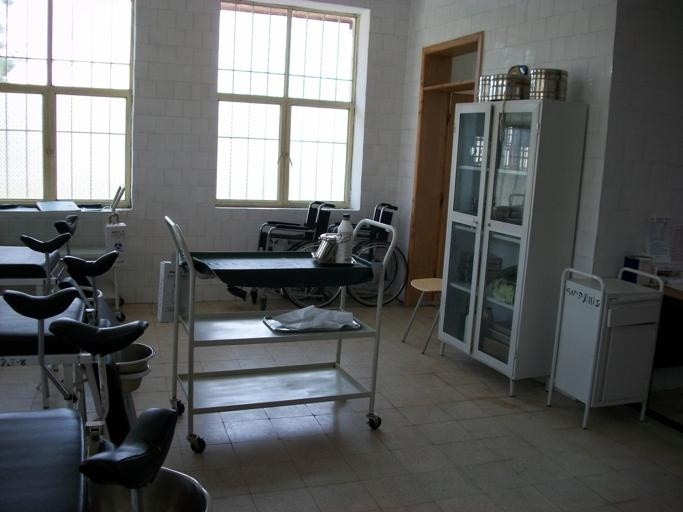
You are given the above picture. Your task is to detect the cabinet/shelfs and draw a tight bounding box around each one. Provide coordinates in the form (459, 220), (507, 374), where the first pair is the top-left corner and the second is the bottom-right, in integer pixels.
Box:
(552, 276), (660, 429)
(436, 100), (589, 397)
(171, 250), (386, 455)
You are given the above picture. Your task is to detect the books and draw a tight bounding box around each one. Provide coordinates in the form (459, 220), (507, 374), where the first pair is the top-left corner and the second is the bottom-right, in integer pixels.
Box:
(462, 253), (517, 363)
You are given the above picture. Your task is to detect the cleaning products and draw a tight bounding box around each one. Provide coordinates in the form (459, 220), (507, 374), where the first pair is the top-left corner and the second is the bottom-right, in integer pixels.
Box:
(337, 213), (354, 263)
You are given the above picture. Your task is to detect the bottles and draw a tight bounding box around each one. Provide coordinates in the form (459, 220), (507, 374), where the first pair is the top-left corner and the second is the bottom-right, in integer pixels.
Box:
(334, 213), (353, 265)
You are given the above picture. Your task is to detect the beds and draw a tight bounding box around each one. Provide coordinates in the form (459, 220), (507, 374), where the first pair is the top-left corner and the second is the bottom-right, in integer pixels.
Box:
(1, 292), (102, 421)
(1, 246), (61, 297)
(1, 408), (92, 512)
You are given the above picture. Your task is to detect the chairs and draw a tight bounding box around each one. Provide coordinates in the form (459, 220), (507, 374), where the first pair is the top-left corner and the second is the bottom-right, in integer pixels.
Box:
(223, 199), (410, 317)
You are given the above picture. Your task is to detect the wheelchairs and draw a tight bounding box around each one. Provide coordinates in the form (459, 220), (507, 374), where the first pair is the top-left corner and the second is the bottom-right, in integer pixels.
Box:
(225, 202), (344, 309)
(327, 203), (408, 308)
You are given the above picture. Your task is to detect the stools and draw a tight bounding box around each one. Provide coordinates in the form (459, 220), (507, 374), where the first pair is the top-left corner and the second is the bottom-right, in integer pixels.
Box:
(401, 277), (443, 355)
